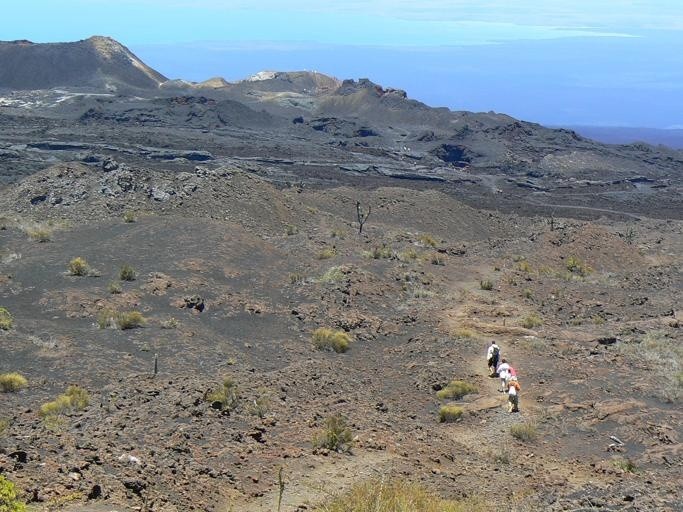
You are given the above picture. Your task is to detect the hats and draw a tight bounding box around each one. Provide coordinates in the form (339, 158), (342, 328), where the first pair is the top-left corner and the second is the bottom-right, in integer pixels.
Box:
(510, 376), (517, 380)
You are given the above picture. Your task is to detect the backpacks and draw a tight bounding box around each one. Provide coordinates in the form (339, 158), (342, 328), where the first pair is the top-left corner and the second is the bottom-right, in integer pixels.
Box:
(509, 386), (516, 397)
(492, 347), (499, 363)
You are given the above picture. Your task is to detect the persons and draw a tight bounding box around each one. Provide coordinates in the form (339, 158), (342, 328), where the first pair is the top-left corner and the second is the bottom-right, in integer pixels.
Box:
(506, 375), (520, 413)
(497, 359), (510, 395)
(486, 340), (500, 376)
(504, 367), (517, 385)
